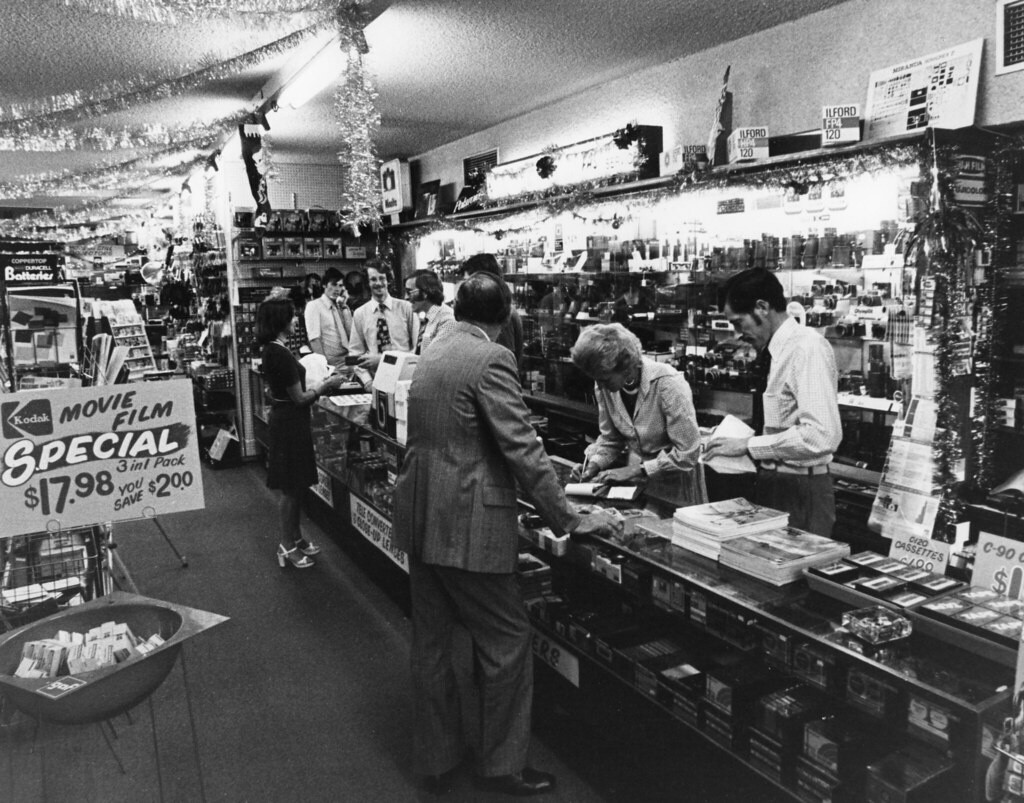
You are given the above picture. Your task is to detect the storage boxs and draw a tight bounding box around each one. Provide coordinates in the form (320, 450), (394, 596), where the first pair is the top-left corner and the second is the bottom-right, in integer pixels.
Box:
(727, 127), (770, 163)
(209, 429), (240, 464)
(822, 105), (860, 146)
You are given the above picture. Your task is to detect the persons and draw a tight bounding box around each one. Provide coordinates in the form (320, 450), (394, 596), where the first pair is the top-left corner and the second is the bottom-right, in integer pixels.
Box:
(701, 268), (843, 538)
(453, 253), (523, 363)
(254, 299), (348, 568)
(304, 267), (353, 382)
(569, 322), (709, 507)
(403, 269), (458, 356)
(389, 270), (624, 797)
(348, 261), (420, 392)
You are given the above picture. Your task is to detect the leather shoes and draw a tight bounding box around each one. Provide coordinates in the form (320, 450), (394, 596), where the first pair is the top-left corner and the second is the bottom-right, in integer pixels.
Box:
(487, 766), (557, 794)
(424, 774), (452, 793)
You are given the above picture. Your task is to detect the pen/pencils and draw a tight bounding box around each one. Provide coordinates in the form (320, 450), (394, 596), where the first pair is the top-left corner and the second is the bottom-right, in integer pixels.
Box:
(349, 373), (354, 381)
(580, 454), (587, 483)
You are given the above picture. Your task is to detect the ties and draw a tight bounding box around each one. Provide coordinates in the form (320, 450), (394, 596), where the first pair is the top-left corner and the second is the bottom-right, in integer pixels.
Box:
(415, 319), (429, 355)
(332, 307), (349, 349)
(376, 304), (392, 353)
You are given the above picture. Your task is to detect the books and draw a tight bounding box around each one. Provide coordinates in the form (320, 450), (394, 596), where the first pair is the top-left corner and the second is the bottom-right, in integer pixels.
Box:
(671, 498), (851, 586)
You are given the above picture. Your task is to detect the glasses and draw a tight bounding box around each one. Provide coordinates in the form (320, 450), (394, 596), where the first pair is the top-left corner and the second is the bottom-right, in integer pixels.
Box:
(406, 289), (419, 294)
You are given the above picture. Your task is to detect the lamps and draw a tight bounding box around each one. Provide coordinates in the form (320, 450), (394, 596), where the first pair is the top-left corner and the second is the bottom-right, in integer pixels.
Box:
(144, 126), (255, 225)
(269, 29), (369, 112)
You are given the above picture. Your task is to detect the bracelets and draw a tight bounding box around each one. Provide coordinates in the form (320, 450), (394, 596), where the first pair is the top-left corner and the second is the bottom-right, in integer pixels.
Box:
(308, 389), (319, 402)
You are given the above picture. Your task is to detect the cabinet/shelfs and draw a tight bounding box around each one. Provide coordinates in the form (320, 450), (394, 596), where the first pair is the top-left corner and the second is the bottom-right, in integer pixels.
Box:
(383, 130), (1024, 514)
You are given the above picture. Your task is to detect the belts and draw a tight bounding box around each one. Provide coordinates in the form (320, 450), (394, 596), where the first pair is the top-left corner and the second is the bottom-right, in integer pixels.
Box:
(761, 460), (829, 475)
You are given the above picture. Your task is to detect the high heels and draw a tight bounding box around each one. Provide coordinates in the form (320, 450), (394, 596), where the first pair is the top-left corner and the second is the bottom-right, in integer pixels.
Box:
(296, 539), (319, 556)
(277, 543), (315, 569)
